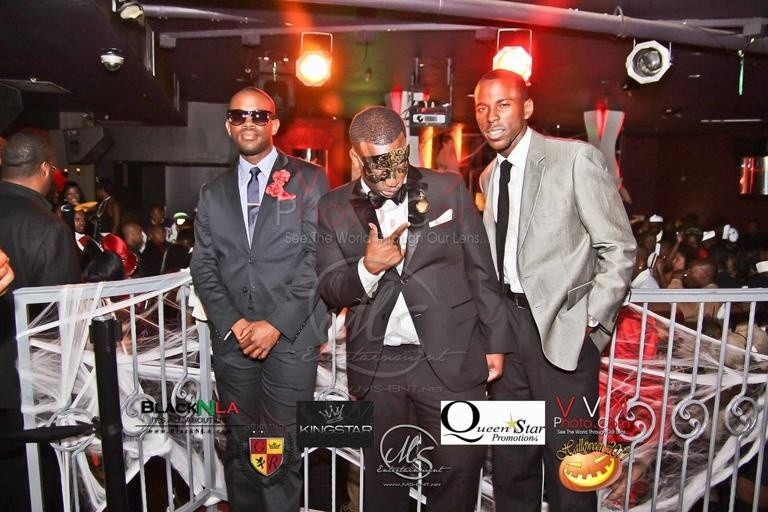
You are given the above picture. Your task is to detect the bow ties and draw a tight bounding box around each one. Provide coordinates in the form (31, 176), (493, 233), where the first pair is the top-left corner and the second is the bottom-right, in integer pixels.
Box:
(368, 184), (407, 209)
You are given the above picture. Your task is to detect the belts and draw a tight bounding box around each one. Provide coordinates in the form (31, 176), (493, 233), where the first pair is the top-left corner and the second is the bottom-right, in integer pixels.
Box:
(507, 292), (532, 312)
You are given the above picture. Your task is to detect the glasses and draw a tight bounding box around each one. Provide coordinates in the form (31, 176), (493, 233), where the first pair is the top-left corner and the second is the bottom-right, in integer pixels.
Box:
(225, 109), (271, 126)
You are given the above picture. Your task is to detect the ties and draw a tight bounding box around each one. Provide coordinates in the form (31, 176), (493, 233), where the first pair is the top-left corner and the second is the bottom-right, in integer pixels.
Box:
(246, 167), (262, 244)
(495, 161), (513, 285)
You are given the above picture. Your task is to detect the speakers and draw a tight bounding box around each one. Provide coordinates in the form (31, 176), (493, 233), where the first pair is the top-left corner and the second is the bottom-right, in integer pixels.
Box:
(63, 126), (114, 164)
(0, 80), (73, 140)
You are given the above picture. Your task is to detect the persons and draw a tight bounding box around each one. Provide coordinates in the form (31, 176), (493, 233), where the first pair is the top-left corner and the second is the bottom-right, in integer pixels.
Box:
(317, 105), (515, 511)
(604, 199), (768, 512)
(189, 87), (332, 512)
(46, 174), (194, 353)
(0, 131), (81, 511)
(473, 70), (638, 512)
(0, 246), (16, 297)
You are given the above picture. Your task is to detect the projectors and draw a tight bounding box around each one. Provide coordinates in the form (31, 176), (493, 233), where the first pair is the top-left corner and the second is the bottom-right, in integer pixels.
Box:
(412, 113), (445, 125)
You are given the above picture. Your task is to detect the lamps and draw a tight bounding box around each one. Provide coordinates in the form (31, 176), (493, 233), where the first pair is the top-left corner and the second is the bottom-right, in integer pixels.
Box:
(492, 29), (534, 88)
(625, 37), (675, 86)
(295, 32), (334, 88)
(111, 0), (145, 32)
